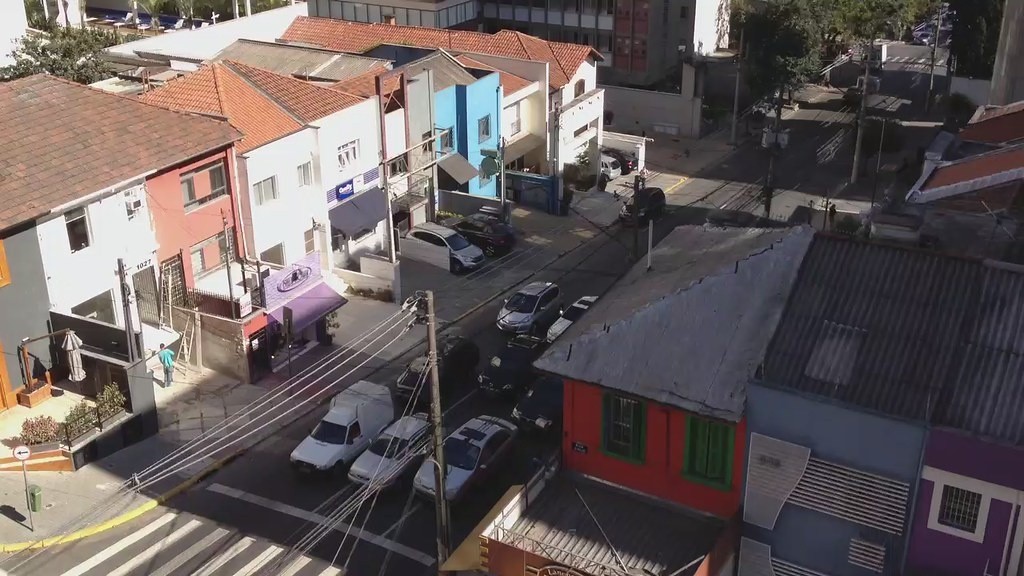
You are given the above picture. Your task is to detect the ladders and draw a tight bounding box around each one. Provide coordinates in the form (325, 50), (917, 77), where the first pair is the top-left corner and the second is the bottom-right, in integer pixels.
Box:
(159, 263), (172, 333)
(174, 315), (195, 375)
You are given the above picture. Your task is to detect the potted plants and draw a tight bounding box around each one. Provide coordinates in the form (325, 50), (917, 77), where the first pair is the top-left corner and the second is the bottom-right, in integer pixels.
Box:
(325, 310), (340, 345)
(19, 381), (126, 452)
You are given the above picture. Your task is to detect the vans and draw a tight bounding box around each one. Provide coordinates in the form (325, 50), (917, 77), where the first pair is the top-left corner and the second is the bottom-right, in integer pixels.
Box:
(288, 381), (395, 480)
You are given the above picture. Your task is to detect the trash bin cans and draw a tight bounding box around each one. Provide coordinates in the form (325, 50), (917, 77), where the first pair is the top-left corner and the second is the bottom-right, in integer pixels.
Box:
(25, 484), (41, 512)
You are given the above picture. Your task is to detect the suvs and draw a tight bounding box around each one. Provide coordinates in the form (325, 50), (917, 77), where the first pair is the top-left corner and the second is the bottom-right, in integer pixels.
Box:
(394, 333), (480, 411)
(446, 213), (517, 260)
(495, 281), (565, 338)
(404, 221), (487, 276)
(600, 148), (638, 182)
(619, 187), (666, 228)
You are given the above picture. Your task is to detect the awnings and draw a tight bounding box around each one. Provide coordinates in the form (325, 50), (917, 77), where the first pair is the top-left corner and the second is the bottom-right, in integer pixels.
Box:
(269, 282), (348, 334)
(438, 153), (479, 190)
(505, 135), (547, 165)
(330, 186), (399, 240)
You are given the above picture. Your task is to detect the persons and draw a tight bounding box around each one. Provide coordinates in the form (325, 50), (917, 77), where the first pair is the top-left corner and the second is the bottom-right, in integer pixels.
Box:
(158, 343), (175, 387)
(640, 167), (649, 190)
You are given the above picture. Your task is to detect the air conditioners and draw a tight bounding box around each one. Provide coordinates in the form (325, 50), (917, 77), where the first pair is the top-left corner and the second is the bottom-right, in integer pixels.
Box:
(125, 197), (142, 211)
(251, 264), (271, 287)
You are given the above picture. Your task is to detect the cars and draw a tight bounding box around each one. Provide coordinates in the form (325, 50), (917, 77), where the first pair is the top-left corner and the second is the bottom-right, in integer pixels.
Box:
(347, 412), (446, 489)
(546, 295), (600, 343)
(475, 334), (548, 401)
(411, 414), (521, 509)
(510, 372), (565, 440)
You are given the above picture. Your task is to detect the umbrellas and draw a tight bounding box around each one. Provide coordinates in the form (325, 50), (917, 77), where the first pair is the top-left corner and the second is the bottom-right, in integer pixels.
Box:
(61, 331), (86, 401)
